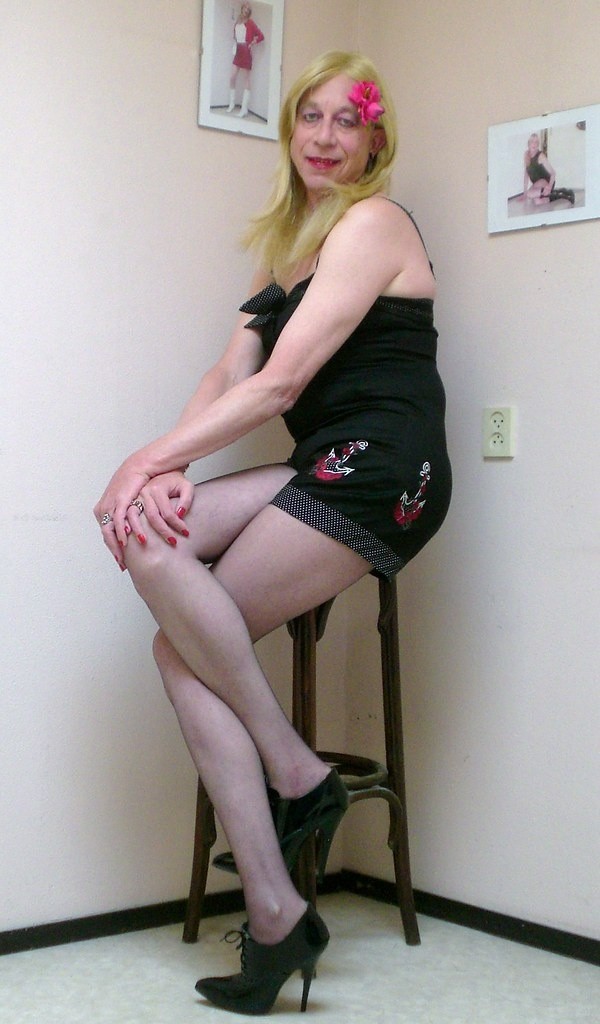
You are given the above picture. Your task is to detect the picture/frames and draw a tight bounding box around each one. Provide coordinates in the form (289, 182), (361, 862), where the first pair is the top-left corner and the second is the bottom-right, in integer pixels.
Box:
(488, 103), (600, 236)
(197, 0), (284, 142)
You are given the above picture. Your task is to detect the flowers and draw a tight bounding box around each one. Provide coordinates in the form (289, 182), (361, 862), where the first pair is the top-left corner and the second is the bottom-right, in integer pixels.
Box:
(349, 79), (385, 125)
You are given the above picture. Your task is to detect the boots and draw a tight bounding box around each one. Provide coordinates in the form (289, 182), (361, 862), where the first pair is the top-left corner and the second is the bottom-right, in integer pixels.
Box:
(541, 187), (574, 204)
(550, 188), (566, 202)
(226, 89), (236, 112)
(238, 90), (251, 117)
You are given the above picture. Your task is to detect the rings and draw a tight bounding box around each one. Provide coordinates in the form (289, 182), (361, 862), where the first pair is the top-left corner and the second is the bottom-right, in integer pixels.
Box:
(101, 513), (114, 524)
(130, 500), (144, 513)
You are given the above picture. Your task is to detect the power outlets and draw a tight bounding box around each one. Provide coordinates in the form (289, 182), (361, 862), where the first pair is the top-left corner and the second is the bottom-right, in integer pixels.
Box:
(483, 407), (513, 459)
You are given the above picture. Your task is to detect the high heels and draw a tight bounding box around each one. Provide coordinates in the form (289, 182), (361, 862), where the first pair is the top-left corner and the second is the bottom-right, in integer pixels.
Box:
(211, 767), (352, 882)
(195, 902), (331, 1016)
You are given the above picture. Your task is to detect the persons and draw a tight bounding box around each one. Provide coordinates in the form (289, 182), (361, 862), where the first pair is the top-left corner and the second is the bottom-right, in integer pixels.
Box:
(517, 133), (575, 205)
(92, 48), (451, 1016)
(226, 1), (265, 117)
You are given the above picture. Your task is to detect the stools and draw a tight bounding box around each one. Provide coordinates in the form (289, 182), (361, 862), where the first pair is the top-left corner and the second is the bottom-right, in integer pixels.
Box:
(183, 572), (420, 943)
